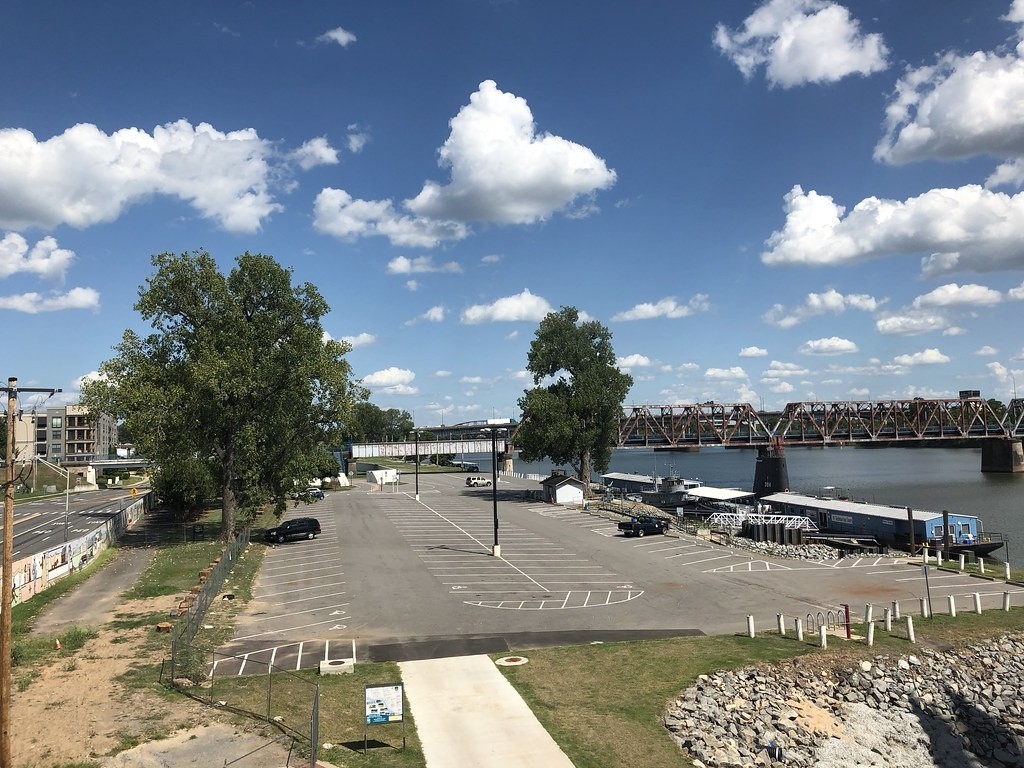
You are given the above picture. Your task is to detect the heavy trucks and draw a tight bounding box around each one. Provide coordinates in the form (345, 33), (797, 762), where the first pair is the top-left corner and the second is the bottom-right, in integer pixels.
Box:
(486, 418), (517, 424)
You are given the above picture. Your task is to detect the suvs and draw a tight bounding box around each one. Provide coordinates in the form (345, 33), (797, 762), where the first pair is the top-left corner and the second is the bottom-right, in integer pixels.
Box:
(466, 476), (492, 487)
(266, 517), (321, 544)
(291, 488), (325, 500)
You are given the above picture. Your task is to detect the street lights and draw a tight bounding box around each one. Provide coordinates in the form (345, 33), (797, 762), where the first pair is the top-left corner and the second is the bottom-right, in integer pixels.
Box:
(19, 419), (28, 460)
(480, 428), (508, 554)
(409, 431), (424, 501)
(32, 455), (69, 542)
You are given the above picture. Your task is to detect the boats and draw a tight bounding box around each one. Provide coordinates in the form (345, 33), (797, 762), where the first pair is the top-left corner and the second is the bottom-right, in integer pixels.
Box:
(451, 462), (478, 468)
(600, 471), (1004, 558)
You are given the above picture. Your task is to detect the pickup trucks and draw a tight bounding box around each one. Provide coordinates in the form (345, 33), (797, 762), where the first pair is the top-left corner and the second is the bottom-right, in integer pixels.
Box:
(616, 515), (668, 538)
